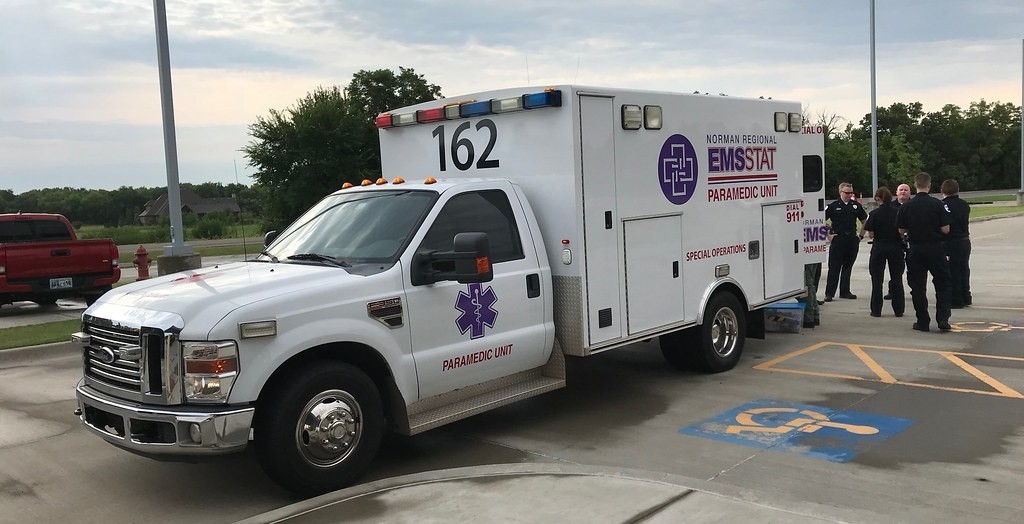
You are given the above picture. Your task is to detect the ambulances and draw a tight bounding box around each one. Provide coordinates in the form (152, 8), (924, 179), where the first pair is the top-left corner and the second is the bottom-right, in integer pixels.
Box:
(73, 80), (827, 497)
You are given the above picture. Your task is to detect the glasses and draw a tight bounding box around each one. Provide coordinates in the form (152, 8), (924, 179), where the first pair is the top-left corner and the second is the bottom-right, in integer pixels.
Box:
(841, 190), (853, 195)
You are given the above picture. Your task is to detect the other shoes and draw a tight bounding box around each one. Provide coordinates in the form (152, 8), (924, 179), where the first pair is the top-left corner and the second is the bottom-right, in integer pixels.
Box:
(839, 293), (857, 299)
(870, 311), (881, 317)
(964, 301), (973, 306)
(885, 293), (893, 299)
(949, 303), (963, 309)
(938, 321), (951, 331)
(824, 294), (832, 302)
(895, 312), (903, 316)
(913, 322), (929, 331)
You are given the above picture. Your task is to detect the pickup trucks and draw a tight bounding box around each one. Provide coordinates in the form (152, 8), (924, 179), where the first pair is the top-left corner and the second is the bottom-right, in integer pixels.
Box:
(0, 213), (122, 309)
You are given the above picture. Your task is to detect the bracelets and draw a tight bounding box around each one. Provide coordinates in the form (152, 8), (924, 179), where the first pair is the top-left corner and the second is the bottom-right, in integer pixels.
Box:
(858, 234), (864, 240)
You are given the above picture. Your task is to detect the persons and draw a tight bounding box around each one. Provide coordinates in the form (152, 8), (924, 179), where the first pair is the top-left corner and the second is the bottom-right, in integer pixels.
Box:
(796, 173), (972, 332)
